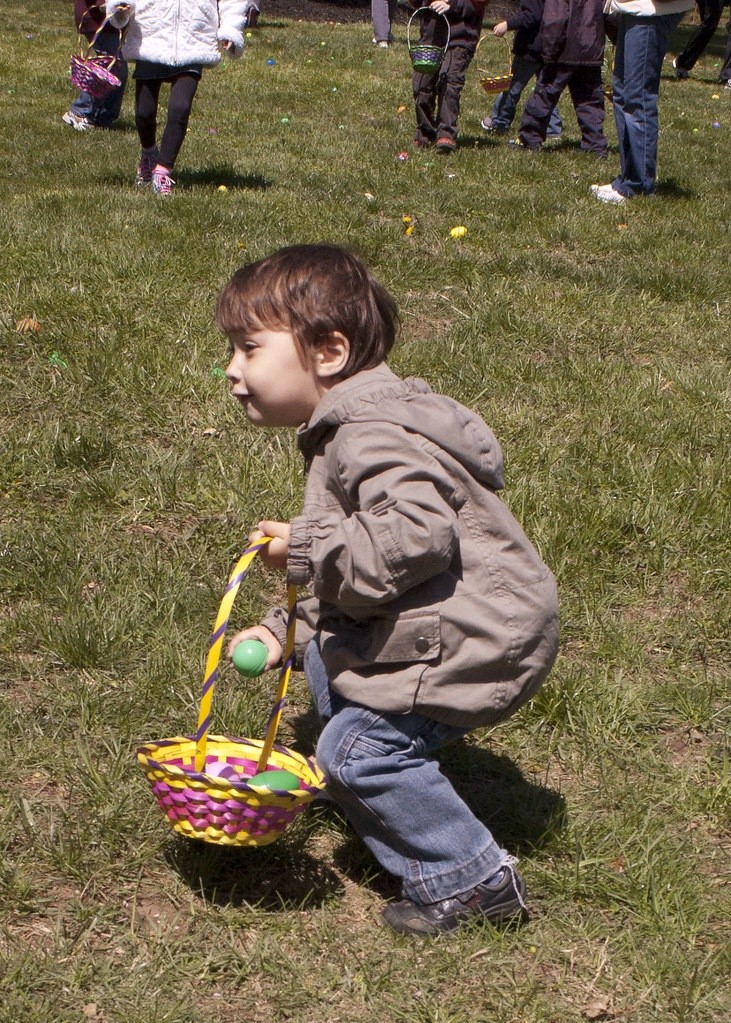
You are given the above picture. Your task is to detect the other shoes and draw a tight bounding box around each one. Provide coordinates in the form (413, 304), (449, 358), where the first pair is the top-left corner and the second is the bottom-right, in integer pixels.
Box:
(372, 37), (377, 43)
(379, 42), (388, 48)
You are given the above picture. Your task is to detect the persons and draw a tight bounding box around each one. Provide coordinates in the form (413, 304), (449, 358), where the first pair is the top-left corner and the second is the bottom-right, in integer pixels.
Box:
(371, 0), (731, 206)
(106, 0), (252, 195)
(62, 0), (129, 133)
(216, 245), (562, 933)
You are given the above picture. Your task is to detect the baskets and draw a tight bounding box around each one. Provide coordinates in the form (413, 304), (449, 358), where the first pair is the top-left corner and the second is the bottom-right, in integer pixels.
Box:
(407, 7), (450, 74)
(135, 535), (328, 847)
(476, 34), (514, 95)
(70, 3), (134, 98)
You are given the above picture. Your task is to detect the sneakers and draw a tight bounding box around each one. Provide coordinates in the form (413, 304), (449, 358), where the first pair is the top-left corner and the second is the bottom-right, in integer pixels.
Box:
(481, 117), (509, 132)
(673, 58), (689, 81)
(591, 184), (626, 206)
(152, 162), (176, 196)
(380, 863), (529, 938)
(136, 145), (160, 184)
(506, 137), (529, 149)
(414, 137), (457, 150)
(722, 79), (731, 88)
(62, 111), (95, 132)
